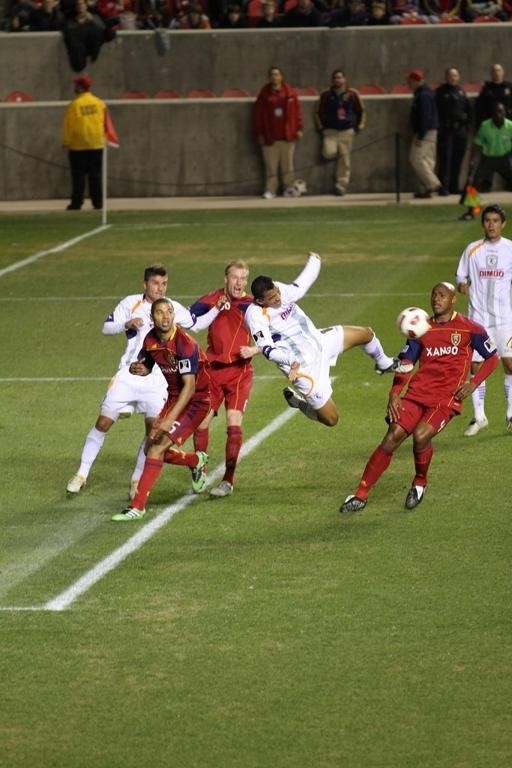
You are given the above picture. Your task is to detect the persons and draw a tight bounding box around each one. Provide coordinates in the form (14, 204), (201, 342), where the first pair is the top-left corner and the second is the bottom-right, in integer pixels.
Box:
(64, 263), (229, 502)
(242, 250), (416, 428)
(338, 280), (501, 515)
(186, 257), (262, 499)
(59, 71), (120, 212)
(310, 70), (368, 195)
(393, 63), (512, 197)
(453, 203), (512, 437)
(106, 298), (213, 525)
(250, 64), (305, 200)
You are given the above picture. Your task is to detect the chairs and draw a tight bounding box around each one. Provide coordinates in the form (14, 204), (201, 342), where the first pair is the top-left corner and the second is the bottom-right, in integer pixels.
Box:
(3, 80), (488, 107)
(0, 0), (511, 31)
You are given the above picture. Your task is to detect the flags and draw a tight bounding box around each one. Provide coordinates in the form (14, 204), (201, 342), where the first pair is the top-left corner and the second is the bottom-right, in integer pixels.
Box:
(103, 106), (120, 150)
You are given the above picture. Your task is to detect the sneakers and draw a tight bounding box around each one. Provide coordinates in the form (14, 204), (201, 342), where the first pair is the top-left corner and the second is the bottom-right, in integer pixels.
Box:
(464, 412), (490, 436)
(65, 202), (83, 211)
(262, 187), (346, 199)
(62, 473), (87, 495)
(414, 185), (474, 221)
(339, 494), (368, 513)
(206, 480), (235, 499)
(403, 478), (429, 511)
(371, 355), (417, 377)
(188, 450), (210, 494)
(281, 386), (304, 410)
(109, 504), (149, 522)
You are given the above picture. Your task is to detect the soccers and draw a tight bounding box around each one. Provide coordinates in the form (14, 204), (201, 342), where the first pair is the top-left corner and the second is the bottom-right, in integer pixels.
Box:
(397, 307), (431, 339)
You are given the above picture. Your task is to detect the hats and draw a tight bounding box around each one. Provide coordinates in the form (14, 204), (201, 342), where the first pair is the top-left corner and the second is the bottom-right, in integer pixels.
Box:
(72, 74), (94, 87)
(403, 69), (424, 82)
